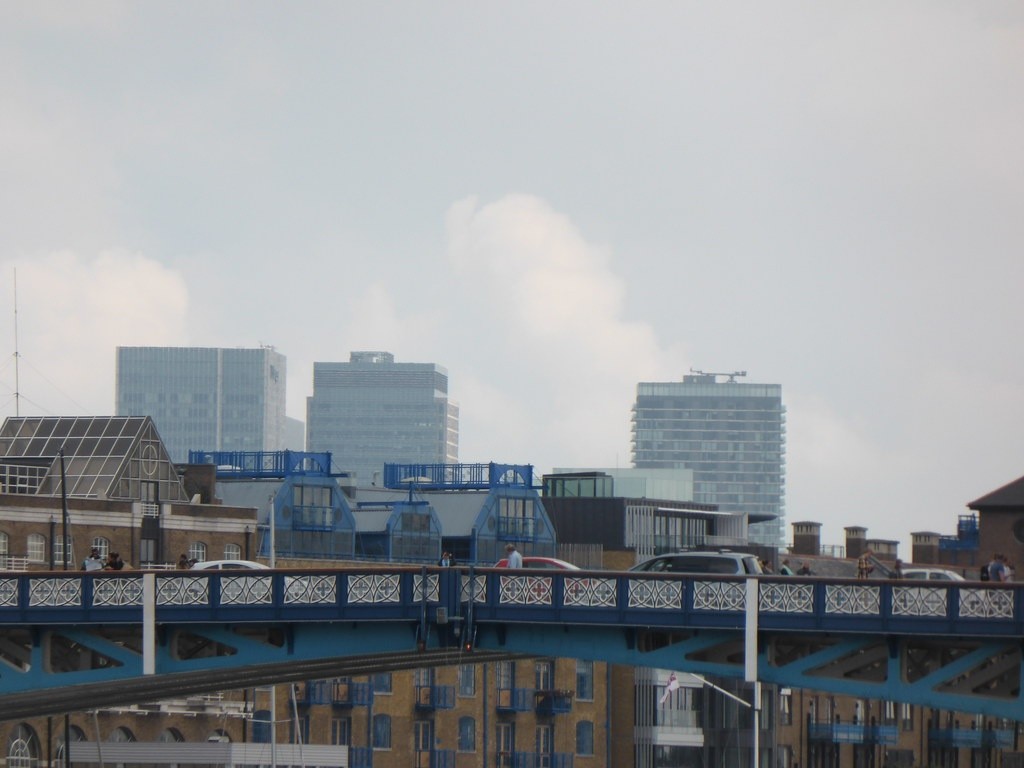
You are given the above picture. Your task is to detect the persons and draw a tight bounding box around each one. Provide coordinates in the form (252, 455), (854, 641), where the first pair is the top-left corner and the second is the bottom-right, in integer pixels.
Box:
(177, 555), (189, 568)
(82, 549), (105, 571)
(506, 543), (522, 569)
(437, 552), (456, 566)
(889, 559), (904, 579)
(762, 559), (774, 574)
(781, 560), (793, 575)
(798, 560), (814, 575)
(859, 549), (874, 578)
(102, 553), (122, 570)
(979, 553), (1016, 583)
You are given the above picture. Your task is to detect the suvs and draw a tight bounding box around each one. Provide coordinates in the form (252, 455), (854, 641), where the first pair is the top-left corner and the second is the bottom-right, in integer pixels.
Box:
(627, 552), (763, 577)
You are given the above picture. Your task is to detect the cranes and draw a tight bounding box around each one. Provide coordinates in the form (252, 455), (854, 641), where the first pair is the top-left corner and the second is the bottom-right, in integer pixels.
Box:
(689, 367), (747, 383)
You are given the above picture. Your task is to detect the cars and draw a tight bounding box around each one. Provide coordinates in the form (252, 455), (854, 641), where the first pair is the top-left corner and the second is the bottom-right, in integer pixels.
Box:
(480, 557), (602, 600)
(903, 569), (986, 602)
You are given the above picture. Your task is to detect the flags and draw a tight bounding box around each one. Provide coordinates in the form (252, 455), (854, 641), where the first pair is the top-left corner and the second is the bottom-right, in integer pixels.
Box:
(660, 671), (678, 705)
